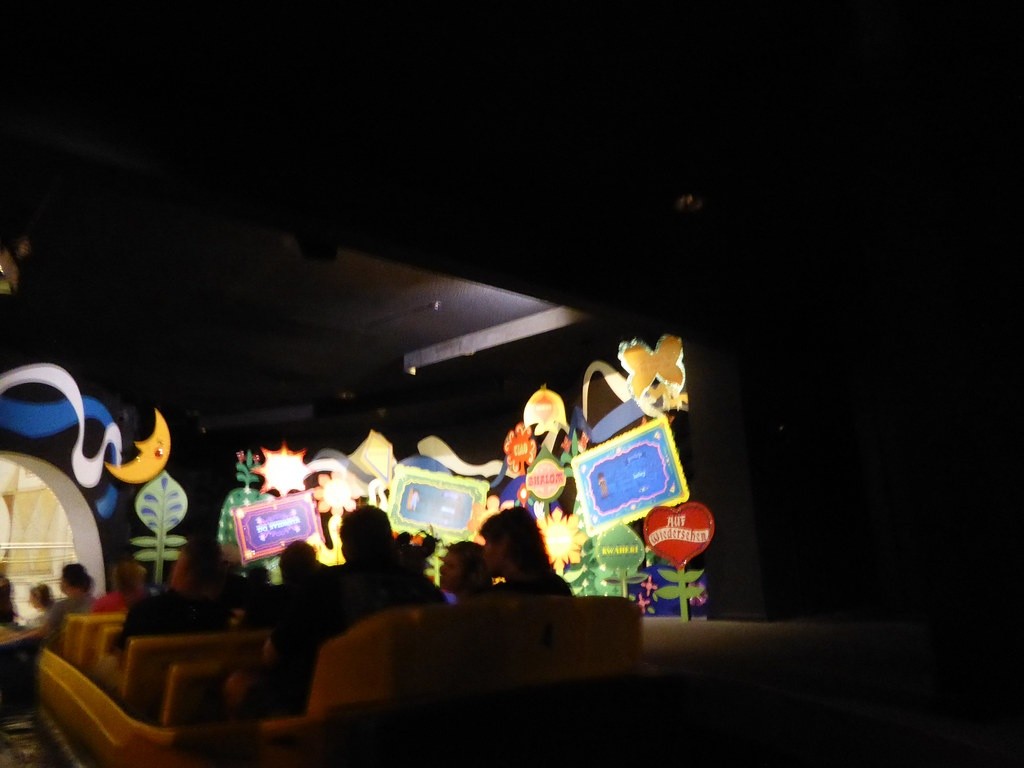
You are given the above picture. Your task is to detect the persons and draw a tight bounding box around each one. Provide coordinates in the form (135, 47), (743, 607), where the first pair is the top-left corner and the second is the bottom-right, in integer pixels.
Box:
(0, 507), (571, 722)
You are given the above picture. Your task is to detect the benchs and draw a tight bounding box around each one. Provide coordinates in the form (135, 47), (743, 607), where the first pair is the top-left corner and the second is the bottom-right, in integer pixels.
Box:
(61, 596), (641, 727)
